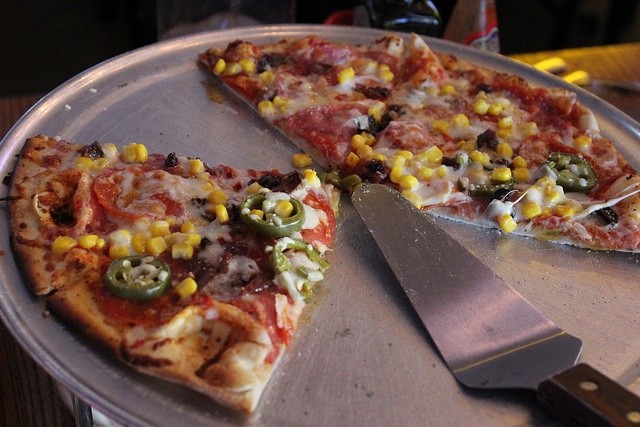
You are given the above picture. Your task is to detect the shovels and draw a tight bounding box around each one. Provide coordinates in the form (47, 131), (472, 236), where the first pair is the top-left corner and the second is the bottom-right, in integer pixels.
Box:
(351, 183), (640, 427)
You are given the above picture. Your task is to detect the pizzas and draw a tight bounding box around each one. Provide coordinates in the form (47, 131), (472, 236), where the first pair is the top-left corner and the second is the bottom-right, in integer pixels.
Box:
(7, 134), (343, 413)
(198, 32), (640, 253)
